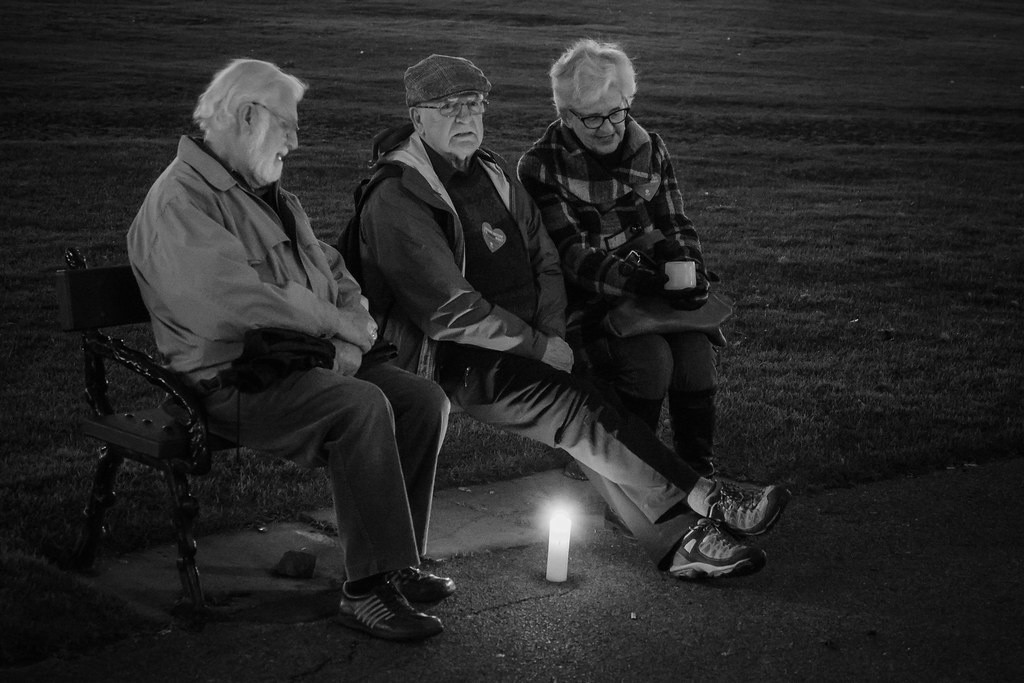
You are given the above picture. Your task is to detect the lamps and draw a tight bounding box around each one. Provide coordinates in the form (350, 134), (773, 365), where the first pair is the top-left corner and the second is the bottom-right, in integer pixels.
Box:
(546, 507), (570, 582)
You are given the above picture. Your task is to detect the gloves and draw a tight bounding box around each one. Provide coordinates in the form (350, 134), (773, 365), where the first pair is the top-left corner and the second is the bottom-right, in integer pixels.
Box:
(643, 259), (711, 310)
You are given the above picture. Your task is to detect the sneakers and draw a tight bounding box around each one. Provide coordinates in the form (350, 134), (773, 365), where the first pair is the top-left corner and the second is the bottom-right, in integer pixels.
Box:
(335, 573), (444, 639)
(382, 565), (457, 602)
(688, 476), (791, 537)
(669, 515), (759, 580)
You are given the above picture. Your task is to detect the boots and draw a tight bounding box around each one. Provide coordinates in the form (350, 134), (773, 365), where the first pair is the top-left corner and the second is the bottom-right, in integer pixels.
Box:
(602, 397), (661, 538)
(667, 386), (716, 475)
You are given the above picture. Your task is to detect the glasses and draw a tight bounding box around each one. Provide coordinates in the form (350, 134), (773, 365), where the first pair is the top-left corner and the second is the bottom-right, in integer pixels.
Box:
(414, 98), (490, 118)
(568, 107), (632, 129)
(252, 99), (300, 140)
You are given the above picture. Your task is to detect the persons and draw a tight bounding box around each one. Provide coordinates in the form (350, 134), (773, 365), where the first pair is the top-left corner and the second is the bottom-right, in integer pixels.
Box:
(516, 37), (720, 544)
(356, 52), (794, 579)
(126, 59), (457, 641)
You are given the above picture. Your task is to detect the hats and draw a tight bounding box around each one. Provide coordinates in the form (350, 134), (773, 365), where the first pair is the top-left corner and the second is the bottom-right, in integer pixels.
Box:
(402, 54), (491, 107)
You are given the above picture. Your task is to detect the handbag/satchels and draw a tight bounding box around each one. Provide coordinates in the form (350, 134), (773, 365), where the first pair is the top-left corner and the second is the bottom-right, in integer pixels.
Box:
(599, 228), (733, 347)
(217, 326), (400, 384)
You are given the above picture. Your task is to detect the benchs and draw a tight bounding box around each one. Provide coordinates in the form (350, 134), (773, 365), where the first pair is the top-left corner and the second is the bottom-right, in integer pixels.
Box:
(53, 247), (465, 621)
